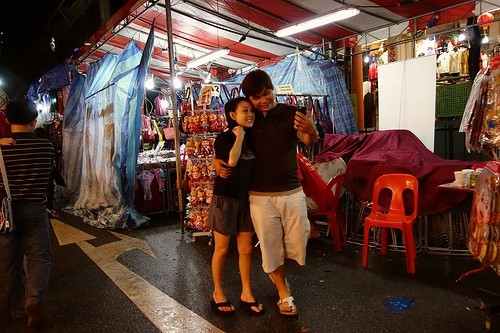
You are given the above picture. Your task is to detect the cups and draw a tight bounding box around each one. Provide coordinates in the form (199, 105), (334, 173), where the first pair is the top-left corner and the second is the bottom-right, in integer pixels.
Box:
(463, 169), (474, 187)
(454, 171), (468, 188)
(470, 171), (480, 189)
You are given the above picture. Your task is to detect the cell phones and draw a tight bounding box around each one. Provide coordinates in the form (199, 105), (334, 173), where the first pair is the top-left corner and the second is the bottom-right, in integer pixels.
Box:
(295, 106), (307, 117)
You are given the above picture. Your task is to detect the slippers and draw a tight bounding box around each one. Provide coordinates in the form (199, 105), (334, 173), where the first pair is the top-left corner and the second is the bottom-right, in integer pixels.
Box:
(277, 295), (298, 316)
(209, 297), (236, 316)
(239, 298), (266, 316)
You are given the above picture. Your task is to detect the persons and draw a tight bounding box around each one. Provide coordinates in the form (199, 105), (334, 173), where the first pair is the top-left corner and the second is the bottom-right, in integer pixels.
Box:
(210, 69), (318, 315)
(206, 97), (265, 317)
(0, 87), (61, 329)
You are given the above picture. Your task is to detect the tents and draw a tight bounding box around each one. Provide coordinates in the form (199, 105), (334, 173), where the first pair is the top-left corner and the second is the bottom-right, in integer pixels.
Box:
(58, 0), (500, 281)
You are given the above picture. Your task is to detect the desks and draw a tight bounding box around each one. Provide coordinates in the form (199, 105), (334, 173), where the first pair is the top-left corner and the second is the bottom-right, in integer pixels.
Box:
(437, 179), (500, 283)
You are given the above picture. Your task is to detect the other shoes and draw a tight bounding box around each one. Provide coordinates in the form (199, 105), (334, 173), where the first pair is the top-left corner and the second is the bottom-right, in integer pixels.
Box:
(12, 307), (27, 321)
(25, 300), (46, 332)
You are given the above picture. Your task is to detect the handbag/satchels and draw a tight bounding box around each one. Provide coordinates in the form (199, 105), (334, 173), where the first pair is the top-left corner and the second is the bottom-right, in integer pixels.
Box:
(143, 84), (209, 150)
(282, 95), (336, 135)
(0, 197), (14, 234)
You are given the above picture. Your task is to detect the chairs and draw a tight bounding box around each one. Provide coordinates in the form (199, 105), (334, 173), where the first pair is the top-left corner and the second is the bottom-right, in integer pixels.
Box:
(303, 151), (349, 253)
(362, 173), (420, 275)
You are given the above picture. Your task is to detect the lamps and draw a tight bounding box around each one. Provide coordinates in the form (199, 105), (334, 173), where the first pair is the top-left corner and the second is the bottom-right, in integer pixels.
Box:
(186, 47), (232, 69)
(273, 5), (360, 38)
